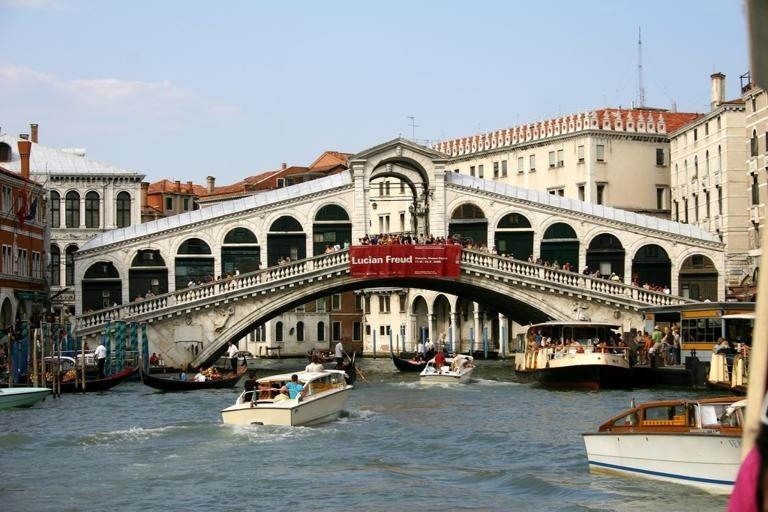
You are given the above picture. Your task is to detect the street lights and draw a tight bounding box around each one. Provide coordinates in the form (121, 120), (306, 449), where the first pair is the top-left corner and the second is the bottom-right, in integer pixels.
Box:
(409, 183), (429, 235)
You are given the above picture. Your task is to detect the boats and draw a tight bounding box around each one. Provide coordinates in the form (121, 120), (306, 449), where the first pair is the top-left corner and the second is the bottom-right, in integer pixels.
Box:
(220, 363), (354, 429)
(0, 318), (140, 411)
(306, 346), (357, 386)
(514, 302), (755, 395)
(581, 396), (747, 495)
(142, 353), (249, 392)
(392, 352), (476, 384)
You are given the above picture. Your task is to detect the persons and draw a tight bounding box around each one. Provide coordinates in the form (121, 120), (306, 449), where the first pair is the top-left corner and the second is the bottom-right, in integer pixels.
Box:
(713, 337), (740, 382)
(84, 342), (89, 354)
(317, 350), (333, 363)
(305, 355), (324, 373)
(727, 392), (768, 511)
(225, 341), (239, 377)
(150, 352), (159, 368)
(88, 307), (93, 313)
(277, 256), (291, 265)
(434, 351), (445, 374)
(696, 295), (704, 301)
(629, 326), (681, 368)
(463, 360), (473, 368)
(92, 342), (107, 379)
(187, 267), (240, 290)
(103, 302), (110, 308)
(279, 374), (304, 399)
(46, 309), (72, 323)
(704, 297), (711, 302)
(358, 233), (514, 259)
(335, 337), (347, 370)
(325, 239), (350, 254)
(528, 255), (670, 294)
(111, 298), (117, 306)
(134, 293), (144, 302)
(193, 367), (206, 383)
(738, 326), (753, 347)
(145, 290), (155, 298)
(528, 328), (629, 354)
(243, 374), (269, 403)
(451, 352), (462, 372)
(409, 338), (435, 365)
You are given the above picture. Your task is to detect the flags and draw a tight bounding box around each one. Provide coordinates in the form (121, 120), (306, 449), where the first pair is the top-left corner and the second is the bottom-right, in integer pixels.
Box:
(11, 181), (44, 231)
